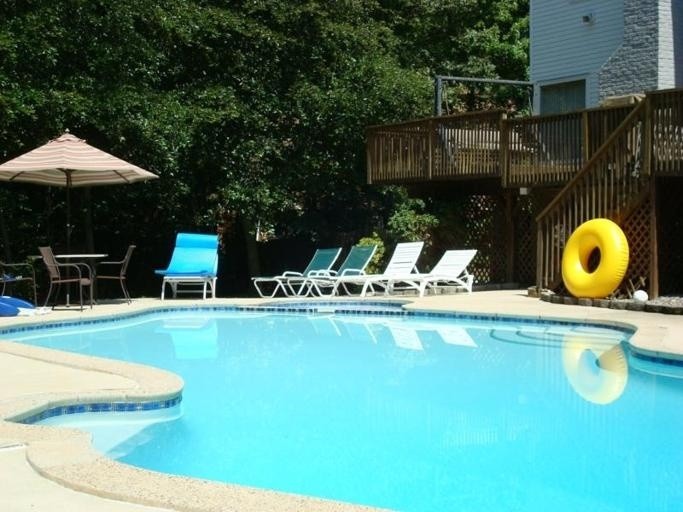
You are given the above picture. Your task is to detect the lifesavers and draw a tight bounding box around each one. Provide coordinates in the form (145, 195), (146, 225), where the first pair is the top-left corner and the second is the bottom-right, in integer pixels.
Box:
(561, 218), (629, 299)
(0, 296), (36, 316)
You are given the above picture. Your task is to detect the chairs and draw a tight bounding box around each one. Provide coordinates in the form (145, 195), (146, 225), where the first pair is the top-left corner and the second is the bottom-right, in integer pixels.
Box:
(270, 316), (478, 349)
(252, 241), (478, 298)
(164, 319), (218, 360)
(156, 233), (220, 300)
(1, 245), (135, 314)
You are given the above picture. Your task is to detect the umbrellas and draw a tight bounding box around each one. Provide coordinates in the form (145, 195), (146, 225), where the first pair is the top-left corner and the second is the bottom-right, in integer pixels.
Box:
(0, 127), (159, 254)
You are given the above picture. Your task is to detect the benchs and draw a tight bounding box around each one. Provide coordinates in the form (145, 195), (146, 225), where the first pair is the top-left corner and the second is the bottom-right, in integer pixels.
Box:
(439, 128), (526, 152)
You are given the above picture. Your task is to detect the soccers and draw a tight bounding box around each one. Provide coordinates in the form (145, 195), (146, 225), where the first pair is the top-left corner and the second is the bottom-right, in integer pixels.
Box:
(632, 290), (648, 304)
